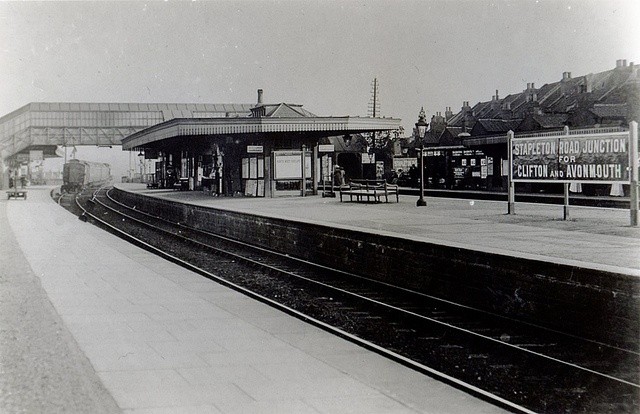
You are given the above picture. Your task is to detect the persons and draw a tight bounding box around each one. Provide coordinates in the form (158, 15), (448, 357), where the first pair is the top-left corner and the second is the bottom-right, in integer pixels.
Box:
(334, 164), (341, 186)
(412, 164), (419, 188)
(340, 167), (345, 185)
(397, 168), (404, 179)
(407, 165), (416, 188)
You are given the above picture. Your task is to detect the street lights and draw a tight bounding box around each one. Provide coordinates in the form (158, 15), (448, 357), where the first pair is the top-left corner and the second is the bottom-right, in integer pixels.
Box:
(415, 116), (429, 206)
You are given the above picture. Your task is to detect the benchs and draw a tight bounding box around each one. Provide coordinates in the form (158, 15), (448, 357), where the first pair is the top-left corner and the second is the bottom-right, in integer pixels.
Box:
(5, 190), (27, 199)
(339, 177), (399, 203)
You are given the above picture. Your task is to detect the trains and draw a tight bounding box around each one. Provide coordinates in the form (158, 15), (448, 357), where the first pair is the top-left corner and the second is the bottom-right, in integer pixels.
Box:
(63, 159), (111, 184)
